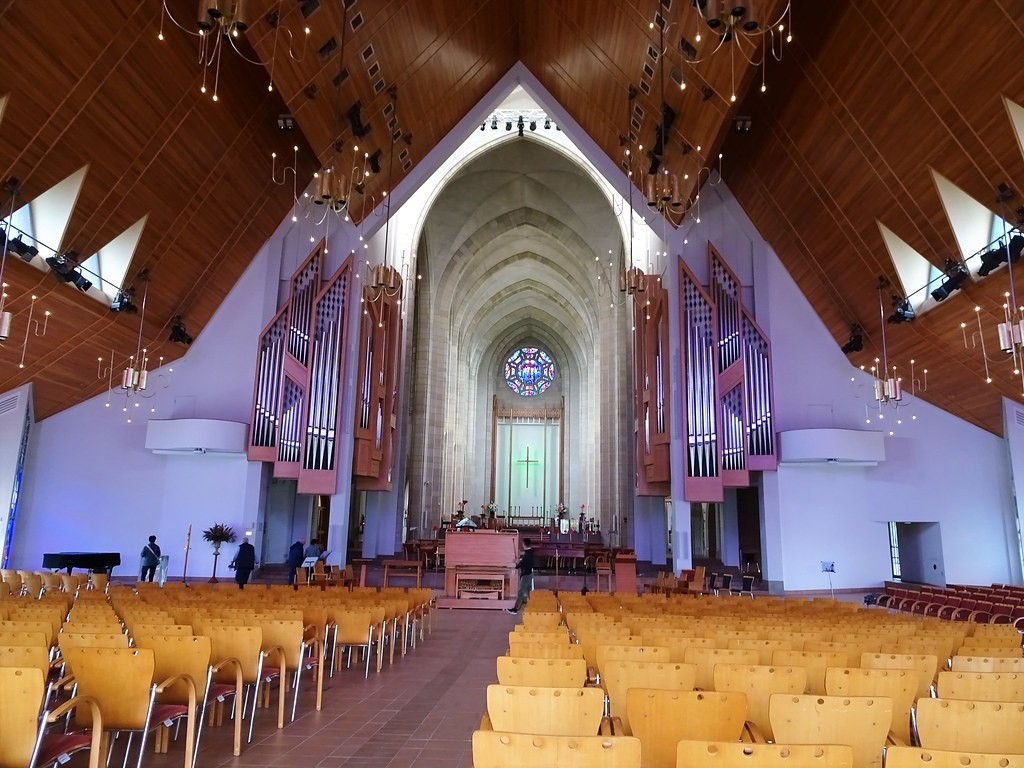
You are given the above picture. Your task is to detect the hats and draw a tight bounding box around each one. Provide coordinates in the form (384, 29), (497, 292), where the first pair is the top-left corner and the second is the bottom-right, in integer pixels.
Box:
(241, 537), (249, 543)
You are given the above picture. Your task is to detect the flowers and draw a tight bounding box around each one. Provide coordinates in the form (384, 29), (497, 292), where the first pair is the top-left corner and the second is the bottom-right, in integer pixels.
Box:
(481, 505), (486, 514)
(458, 502), (464, 510)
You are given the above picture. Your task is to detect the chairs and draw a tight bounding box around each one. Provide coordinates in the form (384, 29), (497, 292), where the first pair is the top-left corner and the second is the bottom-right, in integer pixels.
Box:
(0, 558), (437, 768)
(472, 565), (1024, 768)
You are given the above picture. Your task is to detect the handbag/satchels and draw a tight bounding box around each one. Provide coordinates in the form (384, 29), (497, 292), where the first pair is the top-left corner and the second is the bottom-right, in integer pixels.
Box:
(156, 557), (160, 565)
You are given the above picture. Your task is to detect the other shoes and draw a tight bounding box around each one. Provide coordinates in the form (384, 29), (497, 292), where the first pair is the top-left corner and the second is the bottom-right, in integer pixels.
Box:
(506, 609), (517, 615)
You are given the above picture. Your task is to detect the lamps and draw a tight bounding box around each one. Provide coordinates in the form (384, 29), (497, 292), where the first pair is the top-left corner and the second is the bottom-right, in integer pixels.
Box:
(961, 182), (1024, 400)
(7, 234), (39, 262)
(46, 254), (92, 292)
(850, 270), (926, 435)
(167, 317), (193, 346)
(272, 139), (421, 327)
(97, 267), (174, 423)
(159, 0), (311, 102)
(110, 291), (138, 313)
(841, 324), (863, 355)
(0, 174), (50, 369)
(887, 294), (915, 324)
(931, 259), (970, 302)
(0, 222), (11, 255)
(976, 241), (1004, 276)
(994, 227), (1024, 263)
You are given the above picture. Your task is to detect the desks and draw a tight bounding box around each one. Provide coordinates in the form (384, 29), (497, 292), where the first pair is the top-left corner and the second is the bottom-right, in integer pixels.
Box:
(380, 560), (423, 588)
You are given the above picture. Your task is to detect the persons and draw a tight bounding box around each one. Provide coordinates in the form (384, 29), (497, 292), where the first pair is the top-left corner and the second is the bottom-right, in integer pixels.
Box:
(140, 535), (160, 582)
(228, 537), (255, 589)
(288, 536), (305, 584)
(506, 538), (534, 615)
(304, 539), (321, 580)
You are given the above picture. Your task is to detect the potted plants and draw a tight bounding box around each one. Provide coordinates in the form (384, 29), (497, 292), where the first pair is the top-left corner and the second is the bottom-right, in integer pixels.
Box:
(201, 522), (235, 555)
